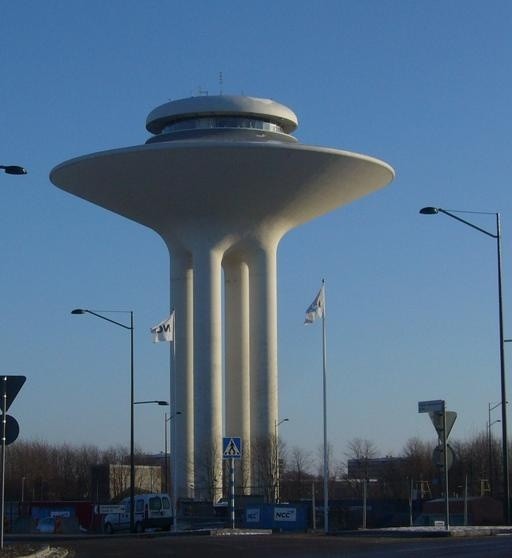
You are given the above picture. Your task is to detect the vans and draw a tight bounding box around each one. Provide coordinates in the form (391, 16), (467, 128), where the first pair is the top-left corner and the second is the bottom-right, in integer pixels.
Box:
(103, 494), (173, 531)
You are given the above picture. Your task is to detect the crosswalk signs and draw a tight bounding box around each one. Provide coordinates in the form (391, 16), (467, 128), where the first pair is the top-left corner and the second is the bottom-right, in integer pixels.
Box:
(222, 437), (242, 460)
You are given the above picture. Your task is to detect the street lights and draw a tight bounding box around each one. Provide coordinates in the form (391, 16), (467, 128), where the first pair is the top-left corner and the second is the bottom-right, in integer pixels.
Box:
(70, 308), (183, 540)
(419, 207), (511, 494)
(272, 413), (290, 507)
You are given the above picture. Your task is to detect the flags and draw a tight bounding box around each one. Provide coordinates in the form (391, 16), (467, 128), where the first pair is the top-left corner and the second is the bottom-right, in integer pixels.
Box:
(303, 282), (326, 326)
(150, 311), (176, 343)
